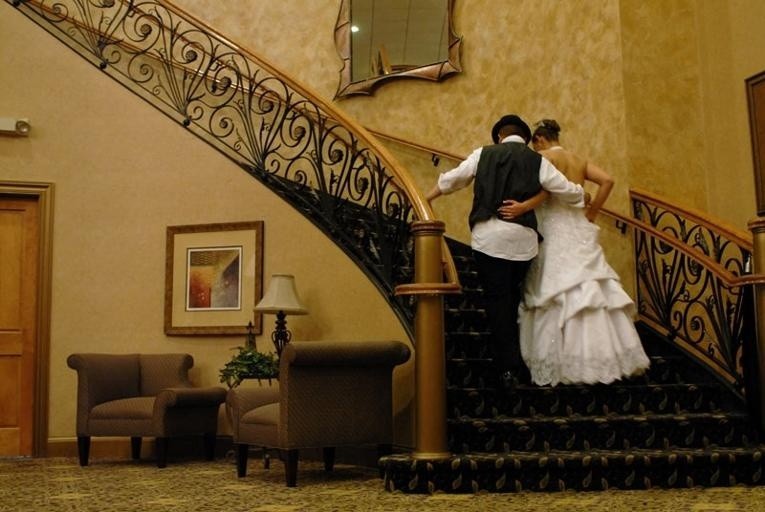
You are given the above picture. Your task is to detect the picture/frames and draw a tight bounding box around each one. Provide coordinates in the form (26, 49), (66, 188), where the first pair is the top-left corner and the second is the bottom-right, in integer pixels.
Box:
(164, 220), (264, 336)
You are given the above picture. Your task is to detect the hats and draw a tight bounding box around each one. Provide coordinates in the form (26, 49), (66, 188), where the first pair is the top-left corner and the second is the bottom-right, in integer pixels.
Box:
(491, 115), (531, 146)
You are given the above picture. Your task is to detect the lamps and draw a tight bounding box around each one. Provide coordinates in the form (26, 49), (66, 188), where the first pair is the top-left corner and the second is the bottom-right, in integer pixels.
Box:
(253, 275), (315, 367)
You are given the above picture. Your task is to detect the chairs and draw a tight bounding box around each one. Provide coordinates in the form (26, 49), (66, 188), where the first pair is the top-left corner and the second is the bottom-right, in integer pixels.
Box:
(224, 341), (412, 488)
(64, 351), (224, 469)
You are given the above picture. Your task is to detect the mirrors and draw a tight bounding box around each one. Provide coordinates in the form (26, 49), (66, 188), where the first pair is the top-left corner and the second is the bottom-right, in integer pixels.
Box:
(334, 0), (465, 101)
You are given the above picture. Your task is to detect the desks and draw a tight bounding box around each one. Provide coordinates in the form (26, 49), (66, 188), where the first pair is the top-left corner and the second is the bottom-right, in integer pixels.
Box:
(224, 372), (287, 470)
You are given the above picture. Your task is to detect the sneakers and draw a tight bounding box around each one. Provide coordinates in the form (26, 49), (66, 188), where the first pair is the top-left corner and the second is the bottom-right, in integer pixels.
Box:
(479, 343), (519, 385)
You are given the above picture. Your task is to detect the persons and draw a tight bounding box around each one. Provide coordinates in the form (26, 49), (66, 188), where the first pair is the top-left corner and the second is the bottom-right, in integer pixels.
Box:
(497, 118), (651, 389)
(424, 115), (592, 391)
